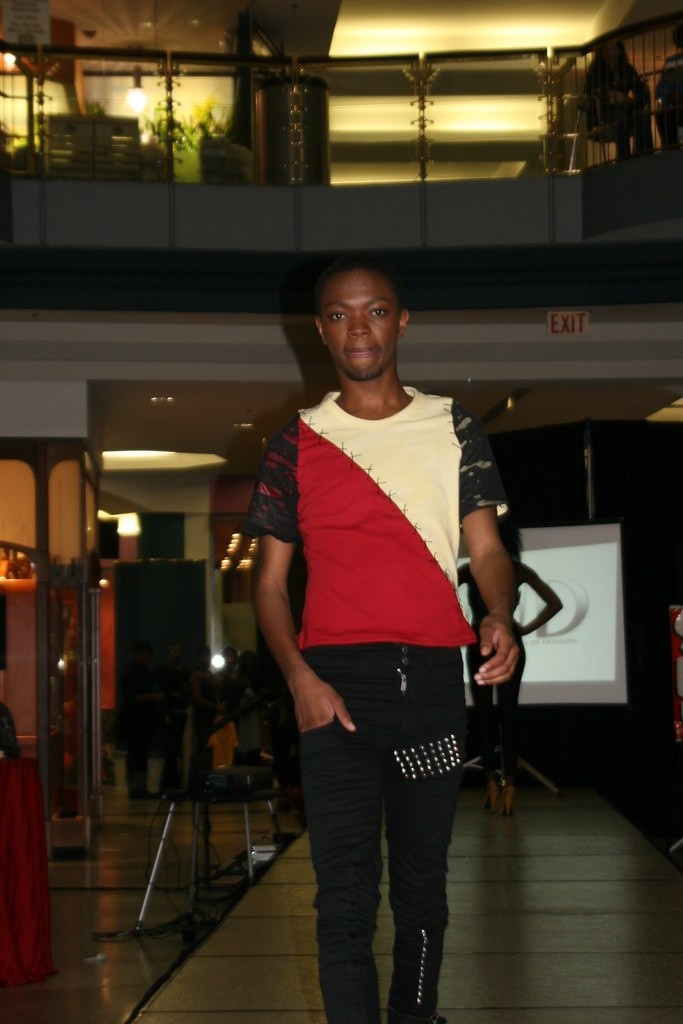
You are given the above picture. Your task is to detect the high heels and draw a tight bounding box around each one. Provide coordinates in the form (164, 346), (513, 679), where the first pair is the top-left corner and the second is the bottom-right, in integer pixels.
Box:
(500, 778), (515, 815)
(483, 783), (500, 813)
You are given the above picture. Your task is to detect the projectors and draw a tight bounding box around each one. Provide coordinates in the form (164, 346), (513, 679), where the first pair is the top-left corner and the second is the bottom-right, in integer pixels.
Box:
(190, 764), (275, 792)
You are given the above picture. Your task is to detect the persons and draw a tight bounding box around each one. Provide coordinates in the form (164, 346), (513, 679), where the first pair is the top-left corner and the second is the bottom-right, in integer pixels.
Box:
(458, 521), (564, 815)
(245, 254), (519, 1024)
(655, 24), (683, 146)
(118, 640), (164, 798)
(157, 640), (301, 814)
(0, 702), (21, 758)
(585, 41), (652, 156)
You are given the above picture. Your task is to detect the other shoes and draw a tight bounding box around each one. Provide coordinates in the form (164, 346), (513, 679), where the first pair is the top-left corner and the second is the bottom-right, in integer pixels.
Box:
(129, 789), (154, 800)
